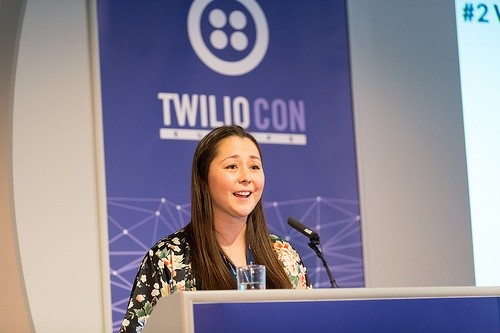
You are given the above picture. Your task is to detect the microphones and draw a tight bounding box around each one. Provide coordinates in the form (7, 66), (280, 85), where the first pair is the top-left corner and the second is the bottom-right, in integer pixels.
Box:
(287, 216), (320, 242)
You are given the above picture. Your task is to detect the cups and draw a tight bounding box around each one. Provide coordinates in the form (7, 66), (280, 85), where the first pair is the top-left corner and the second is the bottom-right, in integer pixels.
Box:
(237, 265), (266, 290)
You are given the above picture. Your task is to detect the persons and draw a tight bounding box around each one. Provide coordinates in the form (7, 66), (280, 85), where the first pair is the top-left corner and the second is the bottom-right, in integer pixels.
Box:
(116, 124), (312, 333)
